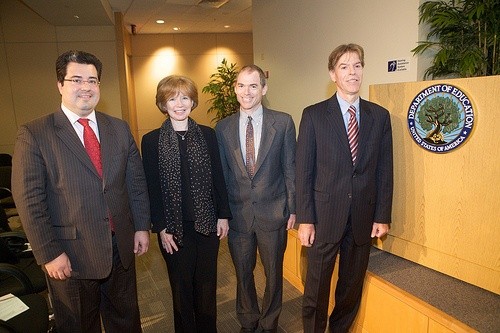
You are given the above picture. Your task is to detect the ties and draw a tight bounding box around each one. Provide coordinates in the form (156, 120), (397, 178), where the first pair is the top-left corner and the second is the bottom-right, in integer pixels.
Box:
(78, 118), (114, 231)
(246, 116), (255, 179)
(347, 105), (359, 167)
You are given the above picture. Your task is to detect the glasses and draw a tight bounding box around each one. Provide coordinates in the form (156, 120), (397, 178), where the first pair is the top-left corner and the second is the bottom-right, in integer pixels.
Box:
(63, 78), (100, 86)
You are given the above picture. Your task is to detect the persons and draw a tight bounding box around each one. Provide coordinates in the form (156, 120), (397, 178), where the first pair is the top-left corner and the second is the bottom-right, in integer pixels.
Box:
(215, 65), (296, 333)
(296, 43), (394, 333)
(141, 74), (233, 333)
(12, 49), (151, 333)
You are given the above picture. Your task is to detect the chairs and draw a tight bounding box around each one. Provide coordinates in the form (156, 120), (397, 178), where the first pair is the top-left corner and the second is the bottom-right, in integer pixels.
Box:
(0, 153), (59, 333)
